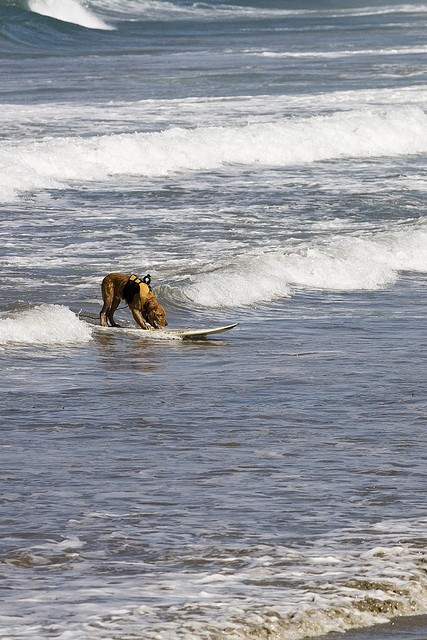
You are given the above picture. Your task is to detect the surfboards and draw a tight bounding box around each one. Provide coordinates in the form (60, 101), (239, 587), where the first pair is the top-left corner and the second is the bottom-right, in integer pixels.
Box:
(88, 322), (240, 336)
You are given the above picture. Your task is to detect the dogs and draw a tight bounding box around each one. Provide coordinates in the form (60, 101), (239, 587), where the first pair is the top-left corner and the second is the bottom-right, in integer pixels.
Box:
(100, 274), (169, 331)
